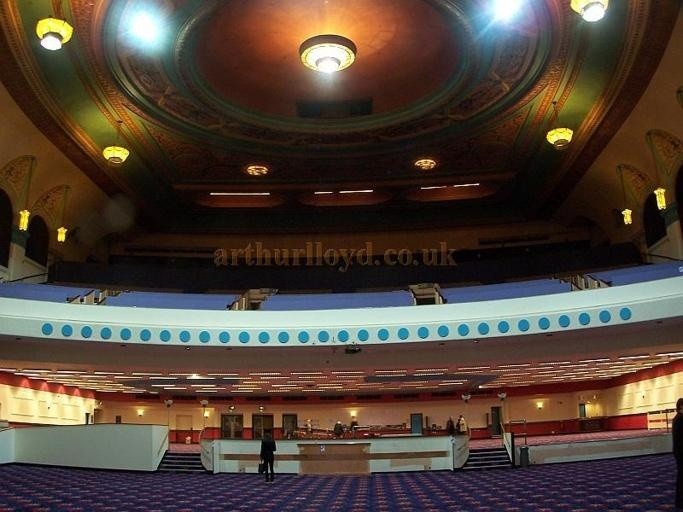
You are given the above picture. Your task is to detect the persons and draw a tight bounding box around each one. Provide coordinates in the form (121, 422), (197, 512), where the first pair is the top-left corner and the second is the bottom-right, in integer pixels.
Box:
(347, 415), (348, 416)
(672, 397), (683, 511)
(447, 414), (455, 436)
(305, 419), (311, 433)
(259, 430), (279, 483)
(334, 415), (359, 435)
(458, 415), (468, 434)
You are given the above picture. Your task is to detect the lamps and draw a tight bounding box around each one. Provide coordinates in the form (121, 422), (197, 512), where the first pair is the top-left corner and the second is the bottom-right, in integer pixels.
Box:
(544, 100), (574, 154)
(98, 118), (131, 167)
(296, 30), (359, 75)
(31, 2), (76, 53)
(567, 0), (610, 25)
(54, 183), (73, 245)
(644, 129), (668, 214)
(615, 164), (635, 228)
(14, 157), (35, 234)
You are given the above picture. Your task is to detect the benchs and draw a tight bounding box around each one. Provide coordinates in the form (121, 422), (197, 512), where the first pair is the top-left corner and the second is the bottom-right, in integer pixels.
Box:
(0, 260), (680, 316)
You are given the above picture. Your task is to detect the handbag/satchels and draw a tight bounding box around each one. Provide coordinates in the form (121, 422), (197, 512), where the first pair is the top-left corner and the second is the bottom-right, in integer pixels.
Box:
(259, 464), (265, 472)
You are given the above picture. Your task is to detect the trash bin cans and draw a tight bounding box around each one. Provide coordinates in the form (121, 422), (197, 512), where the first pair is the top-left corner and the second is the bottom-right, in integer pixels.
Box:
(520, 446), (529, 468)
